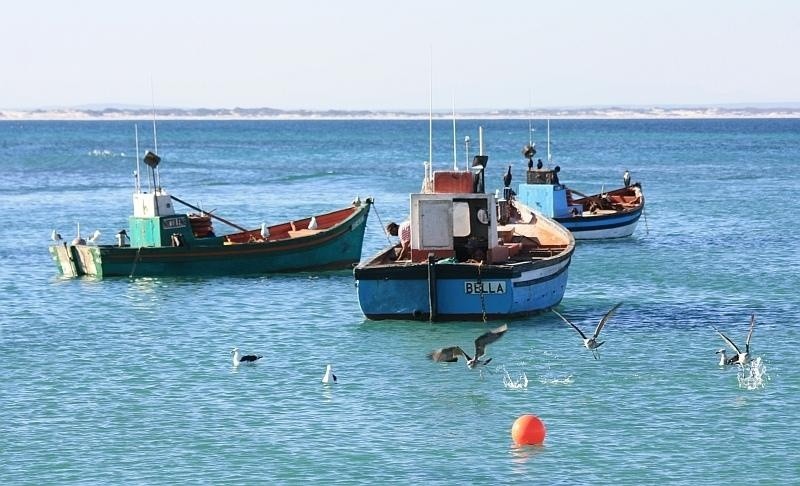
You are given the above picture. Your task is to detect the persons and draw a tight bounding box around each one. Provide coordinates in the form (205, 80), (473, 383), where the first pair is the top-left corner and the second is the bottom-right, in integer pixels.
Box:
(551, 165), (561, 184)
(387, 221), (411, 261)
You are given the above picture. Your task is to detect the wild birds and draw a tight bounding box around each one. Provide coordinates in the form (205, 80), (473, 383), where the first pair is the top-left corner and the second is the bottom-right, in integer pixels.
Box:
(352, 195), (362, 207)
(86, 230), (101, 245)
(322, 363), (338, 384)
(536, 158), (543, 170)
(261, 222), (271, 239)
(431, 323), (508, 372)
(623, 168), (630, 189)
(548, 302), (624, 361)
(51, 229), (63, 246)
(229, 346), (263, 367)
(308, 215), (318, 230)
(589, 200), (598, 214)
(503, 165), (513, 187)
(711, 312), (757, 379)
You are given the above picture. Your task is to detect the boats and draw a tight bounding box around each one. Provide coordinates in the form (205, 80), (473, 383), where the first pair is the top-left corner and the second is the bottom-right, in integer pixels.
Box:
(512, 116), (645, 241)
(47, 120), (372, 280)
(354, 44), (574, 325)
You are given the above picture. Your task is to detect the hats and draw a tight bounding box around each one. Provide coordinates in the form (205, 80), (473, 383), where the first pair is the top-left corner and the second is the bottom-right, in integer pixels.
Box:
(385, 223), (396, 237)
